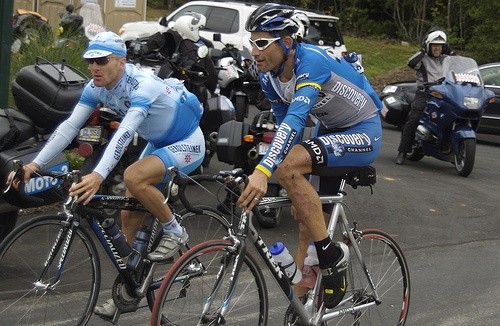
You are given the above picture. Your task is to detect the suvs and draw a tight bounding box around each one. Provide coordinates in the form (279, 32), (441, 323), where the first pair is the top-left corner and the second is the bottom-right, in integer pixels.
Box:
(118, 1), (349, 58)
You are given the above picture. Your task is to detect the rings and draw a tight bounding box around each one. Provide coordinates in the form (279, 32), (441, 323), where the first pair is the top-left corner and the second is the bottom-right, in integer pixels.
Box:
(86, 192), (89, 194)
(254, 198), (259, 200)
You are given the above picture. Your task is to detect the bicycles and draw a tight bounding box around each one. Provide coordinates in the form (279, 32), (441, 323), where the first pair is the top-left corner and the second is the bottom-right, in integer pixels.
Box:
(0, 158), (235, 326)
(149, 168), (412, 326)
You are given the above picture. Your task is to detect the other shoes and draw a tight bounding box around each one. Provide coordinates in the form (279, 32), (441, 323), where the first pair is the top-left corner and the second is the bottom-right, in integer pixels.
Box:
(396, 150), (407, 165)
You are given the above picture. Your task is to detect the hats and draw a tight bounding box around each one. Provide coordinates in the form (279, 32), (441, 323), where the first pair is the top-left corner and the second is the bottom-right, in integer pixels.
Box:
(82, 31), (126, 59)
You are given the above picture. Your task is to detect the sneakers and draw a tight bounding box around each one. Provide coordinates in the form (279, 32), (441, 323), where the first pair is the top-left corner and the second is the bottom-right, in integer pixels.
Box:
(147, 227), (190, 261)
(94, 297), (117, 317)
(320, 241), (350, 309)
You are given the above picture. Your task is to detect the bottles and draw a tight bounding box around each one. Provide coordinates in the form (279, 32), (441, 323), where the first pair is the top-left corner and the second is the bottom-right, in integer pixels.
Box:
(270, 242), (303, 285)
(304, 241), (320, 266)
(127, 225), (151, 270)
(102, 217), (131, 259)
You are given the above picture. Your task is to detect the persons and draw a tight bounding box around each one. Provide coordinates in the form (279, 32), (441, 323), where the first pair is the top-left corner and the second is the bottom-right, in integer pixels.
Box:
(237, 3), (383, 309)
(396, 27), (457, 164)
(6, 31), (205, 316)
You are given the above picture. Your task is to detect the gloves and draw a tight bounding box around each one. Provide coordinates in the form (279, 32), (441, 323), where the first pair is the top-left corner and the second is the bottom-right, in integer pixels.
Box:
(444, 43), (451, 54)
(421, 37), (427, 53)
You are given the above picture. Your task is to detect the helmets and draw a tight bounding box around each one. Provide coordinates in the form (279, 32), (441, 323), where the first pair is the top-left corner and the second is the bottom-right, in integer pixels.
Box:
(174, 11), (206, 43)
(425, 26), (448, 54)
(244, 2), (311, 40)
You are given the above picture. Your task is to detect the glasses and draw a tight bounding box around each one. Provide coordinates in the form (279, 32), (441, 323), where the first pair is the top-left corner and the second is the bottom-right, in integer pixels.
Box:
(84, 56), (121, 66)
(248, 36), (285, 50)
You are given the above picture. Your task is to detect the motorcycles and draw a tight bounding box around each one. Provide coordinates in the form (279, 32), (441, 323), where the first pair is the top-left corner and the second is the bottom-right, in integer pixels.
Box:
(57, 7), (85, 38)
(0, 15), (320, 238)
(398, 56), (499, 178)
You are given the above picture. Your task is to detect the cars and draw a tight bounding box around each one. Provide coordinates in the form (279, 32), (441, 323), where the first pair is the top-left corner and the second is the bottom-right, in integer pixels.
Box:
(377, 61), (500, 136)
